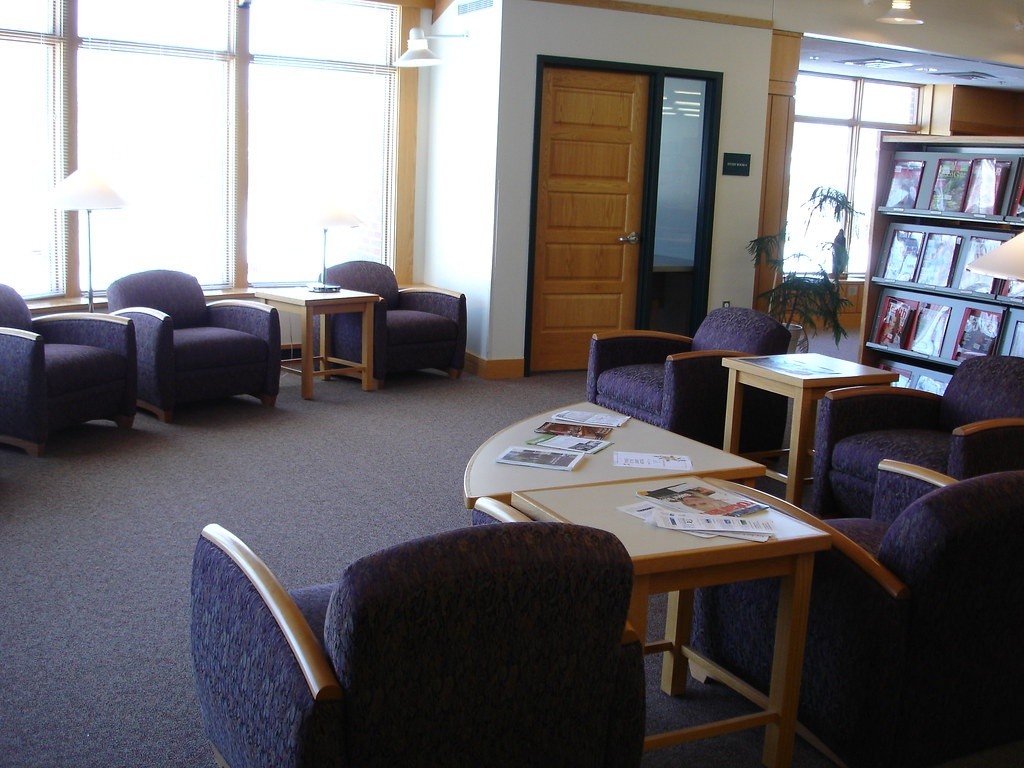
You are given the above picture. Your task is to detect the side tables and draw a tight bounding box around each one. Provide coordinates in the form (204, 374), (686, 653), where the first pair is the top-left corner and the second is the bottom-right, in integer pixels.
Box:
(722, 352), (900, 509)
(254, 286), (380, 400)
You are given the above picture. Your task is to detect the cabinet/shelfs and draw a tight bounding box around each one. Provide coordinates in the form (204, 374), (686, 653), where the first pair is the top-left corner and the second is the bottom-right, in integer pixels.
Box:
(859, 129), (1024, 396)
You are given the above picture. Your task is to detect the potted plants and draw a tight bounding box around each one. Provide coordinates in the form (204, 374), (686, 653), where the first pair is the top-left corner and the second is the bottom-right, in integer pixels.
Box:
(745, 185), (864, 355)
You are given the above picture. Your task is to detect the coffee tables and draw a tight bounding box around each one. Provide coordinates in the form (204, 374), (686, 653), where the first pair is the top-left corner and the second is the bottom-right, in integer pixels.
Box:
(510, 474), (832, 768)
(463, 401), (767, 491)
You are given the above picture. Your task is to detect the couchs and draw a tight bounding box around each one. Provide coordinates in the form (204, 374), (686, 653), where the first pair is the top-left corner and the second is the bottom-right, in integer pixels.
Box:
(0, 261), (1024, 768)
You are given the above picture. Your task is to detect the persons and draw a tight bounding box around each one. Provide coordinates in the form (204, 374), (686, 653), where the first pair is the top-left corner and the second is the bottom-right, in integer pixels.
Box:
(648, 490), (729, 515)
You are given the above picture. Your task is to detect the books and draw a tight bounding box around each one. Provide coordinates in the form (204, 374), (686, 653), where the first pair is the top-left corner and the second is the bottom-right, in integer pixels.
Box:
(528, 435), (614, 455)
(553, 408), (631, 427)
(636, 483), (770, 520)
(496, 446), (585, 471)
(875, 152), (1024, 396)
(535, 421), (612, 439)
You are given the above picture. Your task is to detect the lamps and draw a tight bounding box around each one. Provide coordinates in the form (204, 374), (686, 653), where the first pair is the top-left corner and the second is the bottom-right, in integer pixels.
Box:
(42, 164), (122, 313)
(392, 26), (472, 68)
(875, 0), (925, 25)
